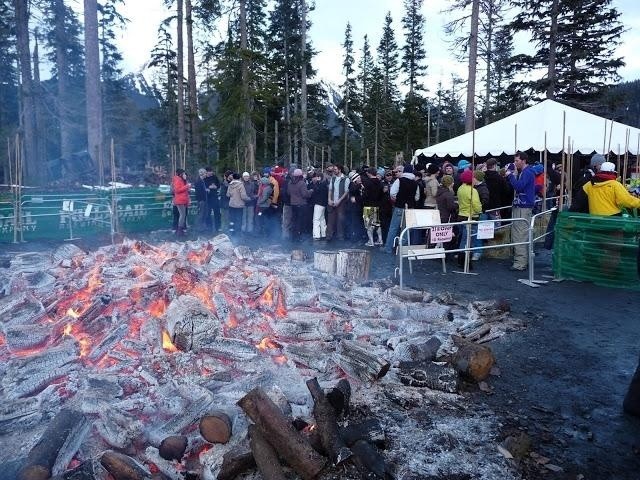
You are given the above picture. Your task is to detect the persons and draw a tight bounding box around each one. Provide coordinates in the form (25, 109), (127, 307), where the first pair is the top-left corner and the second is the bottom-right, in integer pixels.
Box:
(171, 150), (640, 273)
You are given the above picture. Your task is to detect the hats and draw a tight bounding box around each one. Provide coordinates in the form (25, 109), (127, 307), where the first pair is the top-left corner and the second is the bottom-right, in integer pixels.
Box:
(294, 169), (302, 176)
(458, 160), (473, 182)
(591, 154), (617, 173)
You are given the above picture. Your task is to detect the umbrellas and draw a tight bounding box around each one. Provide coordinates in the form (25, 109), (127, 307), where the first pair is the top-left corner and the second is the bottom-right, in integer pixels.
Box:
(415, 97), (639, 162)
